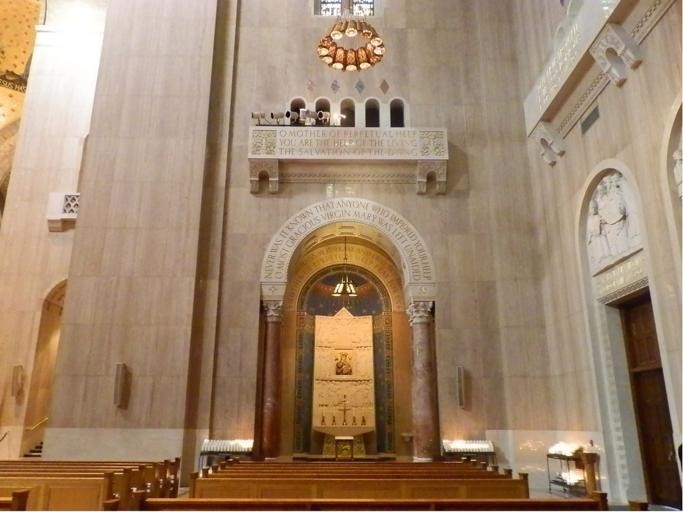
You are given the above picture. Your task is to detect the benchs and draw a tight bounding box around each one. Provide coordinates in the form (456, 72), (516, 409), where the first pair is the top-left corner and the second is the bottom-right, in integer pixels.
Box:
(145, 454), (608, 510)
(0, 458), (181, 510)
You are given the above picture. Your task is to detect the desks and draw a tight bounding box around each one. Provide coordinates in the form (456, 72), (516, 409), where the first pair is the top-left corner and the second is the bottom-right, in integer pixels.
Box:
(334, 435), (354, 458)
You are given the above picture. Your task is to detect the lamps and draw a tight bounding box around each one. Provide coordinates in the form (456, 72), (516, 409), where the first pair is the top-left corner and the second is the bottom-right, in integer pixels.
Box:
(249, 109), (331, 127)
(312, 0), (385, 72)
(330, 236), (359, 298)
